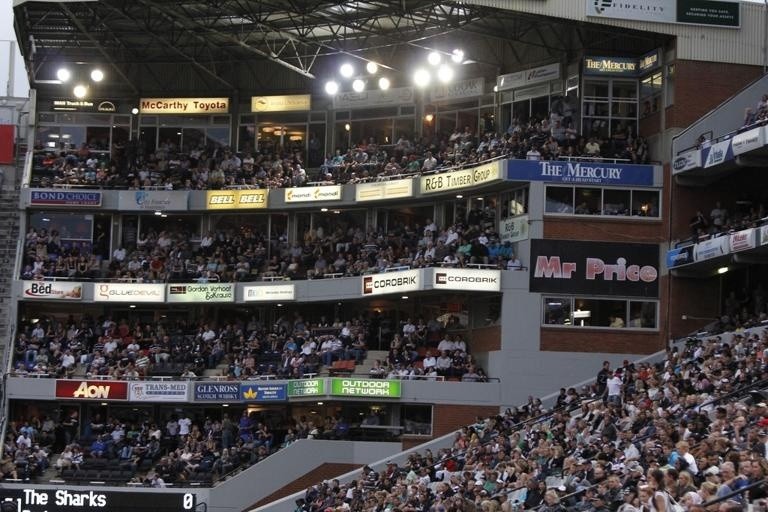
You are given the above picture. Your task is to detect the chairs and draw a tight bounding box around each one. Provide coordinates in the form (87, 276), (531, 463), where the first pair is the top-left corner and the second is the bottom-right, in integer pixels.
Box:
(154, 354), (184, 375)
(256, 350), (279, 374)
(172, 472), (204, 485)
(62, 459), (152, 487)
(327, 359), (355, 378)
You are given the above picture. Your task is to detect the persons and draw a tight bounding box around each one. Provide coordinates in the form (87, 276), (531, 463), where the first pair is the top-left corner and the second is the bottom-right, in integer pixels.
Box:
(1, 97), (768, 510)
